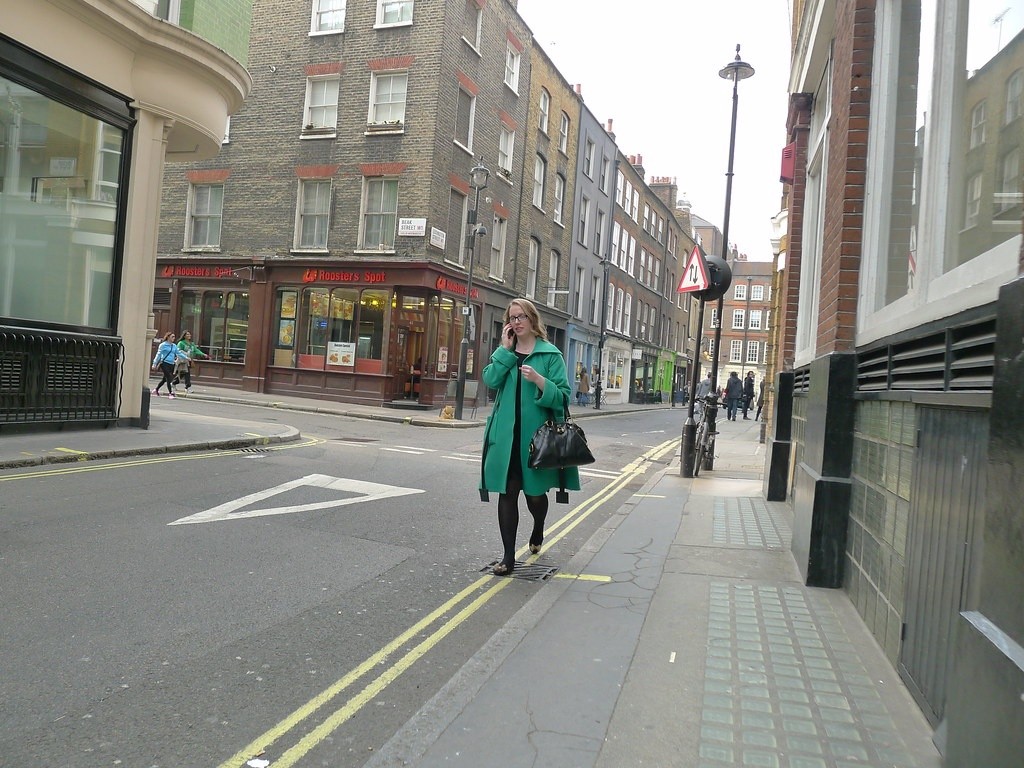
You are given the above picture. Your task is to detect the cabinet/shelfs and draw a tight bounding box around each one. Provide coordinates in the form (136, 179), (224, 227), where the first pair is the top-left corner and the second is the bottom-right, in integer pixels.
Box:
(211, 324), (248, 364)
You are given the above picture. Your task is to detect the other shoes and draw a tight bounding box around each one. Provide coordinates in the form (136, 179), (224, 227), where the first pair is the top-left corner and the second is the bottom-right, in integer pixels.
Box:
(171, 383), (178, 392)
(184, 386), (193, 393)
(755, 418), (757, 421)
(728, 416), (731, 421)
(152, 388), (161, 397)
(732, 417), (736, 421)
(529, 535), (543, 553)
(168, 391), (176, 400)
(743, 416), (751, 420)
(492, 558), (516, 575)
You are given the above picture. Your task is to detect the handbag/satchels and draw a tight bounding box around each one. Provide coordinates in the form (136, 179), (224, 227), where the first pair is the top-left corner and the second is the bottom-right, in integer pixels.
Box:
(576, 391), (579, 398)
(527, 392), (595, 468)
(156, 361), (163, 371)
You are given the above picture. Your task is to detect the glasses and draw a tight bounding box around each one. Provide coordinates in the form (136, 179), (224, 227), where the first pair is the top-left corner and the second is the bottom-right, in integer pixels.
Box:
(170, 335), (175, 338)
(507, 313), (528, 323)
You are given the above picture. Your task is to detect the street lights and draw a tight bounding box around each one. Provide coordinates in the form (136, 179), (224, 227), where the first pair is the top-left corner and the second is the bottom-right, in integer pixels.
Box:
(702, 53), (755, 469)
(455, 166), (490, 420)
(593, 253), (611, 410)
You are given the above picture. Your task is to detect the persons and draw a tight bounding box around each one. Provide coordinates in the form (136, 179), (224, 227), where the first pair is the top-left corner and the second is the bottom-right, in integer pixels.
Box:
(724, 371), (743, 421)
(171, 330), (212, 393)
(683, 380), (692, 403)
(743, 371), (754, 420)
(576, 367), (590, 407)
(694, 371), (712, 421)
(755, 375), (766, 421)
(479, 298), (581, 575)
(151, 331), (191, 400)
